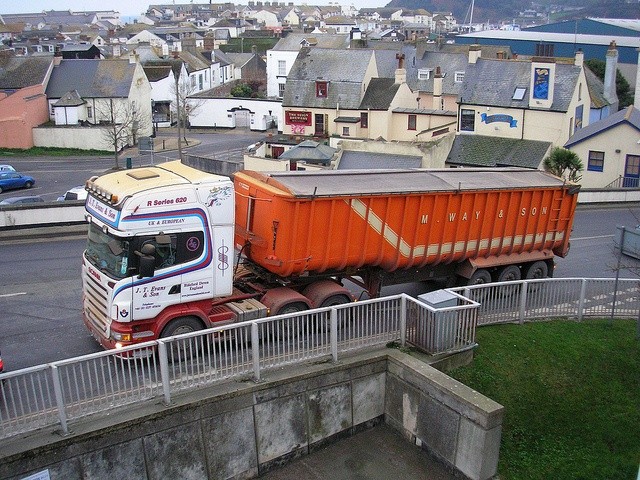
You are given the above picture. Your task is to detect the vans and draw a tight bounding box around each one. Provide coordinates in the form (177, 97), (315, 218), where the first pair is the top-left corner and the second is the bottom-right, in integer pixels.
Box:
(0, 196), (44, 205)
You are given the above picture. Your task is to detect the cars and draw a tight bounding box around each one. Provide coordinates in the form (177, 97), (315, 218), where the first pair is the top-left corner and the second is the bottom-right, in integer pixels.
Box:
(0, 171), (35, 194)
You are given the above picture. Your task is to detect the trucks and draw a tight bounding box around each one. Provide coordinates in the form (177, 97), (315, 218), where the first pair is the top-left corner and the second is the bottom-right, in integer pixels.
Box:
(81, 166), (581, 363)
